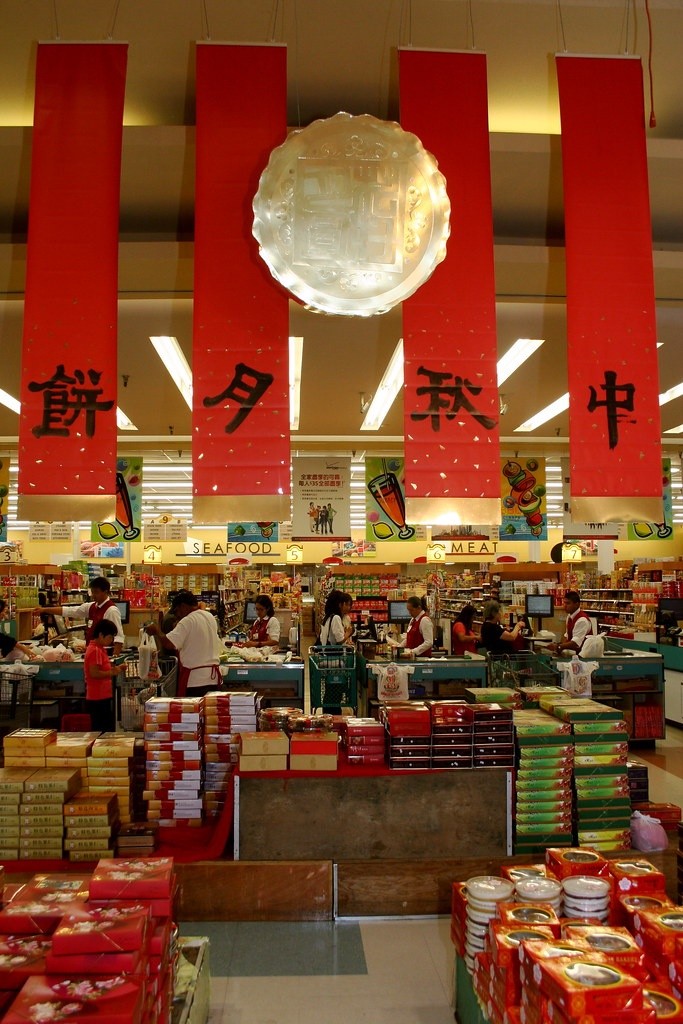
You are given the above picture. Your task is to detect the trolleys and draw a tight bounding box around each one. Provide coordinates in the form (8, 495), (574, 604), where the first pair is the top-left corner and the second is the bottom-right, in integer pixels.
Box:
(116, 655), (179, 731)
(0, 670), (38, 730)
(306, 645), (360, 717)
(485, 648), (561, 688)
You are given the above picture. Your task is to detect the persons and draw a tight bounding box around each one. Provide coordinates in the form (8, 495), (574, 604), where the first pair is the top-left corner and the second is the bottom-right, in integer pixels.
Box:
(0, 599), (36, 662)
(452, 605), (482, 655)
(242, 595), (280, 709)
(397, 595), (434, 697)
(32, 576), (124, 732)
(480, 600), (526, 689)
(143, 589), (223, 697)
(307, 502), (337, 535)
(548, 591), (593, 658)
(320, 589), (355, 716)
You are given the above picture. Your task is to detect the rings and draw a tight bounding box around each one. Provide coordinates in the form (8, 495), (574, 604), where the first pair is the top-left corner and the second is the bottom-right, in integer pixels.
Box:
(30, 655), (31, 657)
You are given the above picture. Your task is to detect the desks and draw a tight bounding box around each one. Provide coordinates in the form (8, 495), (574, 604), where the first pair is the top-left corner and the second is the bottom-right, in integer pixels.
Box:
(172, 935), (212, 1024)
(367, 663), (487, 687)
(224, 663), (305, 697)
(555, 648), (664, 693)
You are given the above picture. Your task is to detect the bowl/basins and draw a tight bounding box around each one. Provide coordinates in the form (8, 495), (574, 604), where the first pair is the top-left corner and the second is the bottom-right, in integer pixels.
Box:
(463, 874), (611, 976)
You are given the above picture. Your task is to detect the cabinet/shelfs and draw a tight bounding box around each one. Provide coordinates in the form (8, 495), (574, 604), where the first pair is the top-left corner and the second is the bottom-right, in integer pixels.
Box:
(0, 562), (683, 650)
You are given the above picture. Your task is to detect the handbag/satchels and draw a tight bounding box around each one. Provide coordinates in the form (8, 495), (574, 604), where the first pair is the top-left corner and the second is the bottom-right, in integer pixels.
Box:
(475, 638), (484, 649)
(579, 632), (607, 659)
(312, 624), (324, 656)
(324, 614), (344, 656)
(138, 629), (162, 680)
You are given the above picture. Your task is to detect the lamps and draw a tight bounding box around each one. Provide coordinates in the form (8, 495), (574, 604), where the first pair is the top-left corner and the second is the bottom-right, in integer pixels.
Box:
(497, 338), (545, 389)
(658, 382), (683, 406)
(662, 424), (683, 434)
(359, 336), (404, 431)
(149, 334), (193, 412)
(0, 388), (22, 416)
(512, 342), (665, 433)
(115, 407), (139, 431)
(287, 336), (304, 431)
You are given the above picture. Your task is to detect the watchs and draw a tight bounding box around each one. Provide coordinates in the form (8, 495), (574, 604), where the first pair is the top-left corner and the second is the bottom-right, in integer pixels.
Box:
(557, 644), (561, 651)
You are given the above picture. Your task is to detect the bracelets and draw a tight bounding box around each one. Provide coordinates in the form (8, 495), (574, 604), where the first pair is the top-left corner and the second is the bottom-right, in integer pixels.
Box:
(515, 625), (520, 630)
(257, 642), (262, 648)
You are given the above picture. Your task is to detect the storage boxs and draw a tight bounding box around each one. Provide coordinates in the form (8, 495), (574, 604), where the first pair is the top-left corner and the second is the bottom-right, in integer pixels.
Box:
(0, 683), (683, 1024)
(621, 706), (664, 739)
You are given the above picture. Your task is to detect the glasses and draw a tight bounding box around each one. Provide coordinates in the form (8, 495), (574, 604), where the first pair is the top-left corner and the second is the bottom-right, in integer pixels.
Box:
(254, 607), (266, 612)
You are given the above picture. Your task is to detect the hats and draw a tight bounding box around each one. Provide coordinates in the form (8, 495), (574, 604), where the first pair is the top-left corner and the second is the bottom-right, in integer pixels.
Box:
(168, 591), (198, 615)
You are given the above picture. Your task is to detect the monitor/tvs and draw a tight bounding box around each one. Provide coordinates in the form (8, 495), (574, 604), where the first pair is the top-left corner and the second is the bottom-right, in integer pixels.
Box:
(524, 594), (554, 618)
(522, 614), (533, 636)
(388, 600), (412, 624)
(243, 601), (258, 624)
(366, 615), (377, 640)
(113, 600), (129, 624)
(657, 597), (683, 621)
